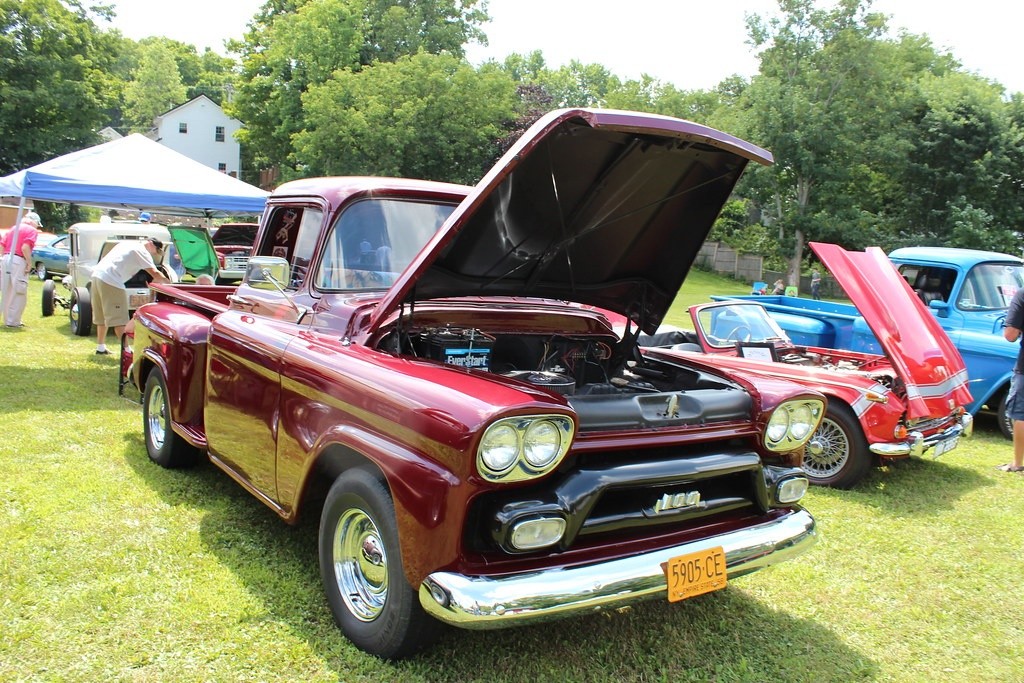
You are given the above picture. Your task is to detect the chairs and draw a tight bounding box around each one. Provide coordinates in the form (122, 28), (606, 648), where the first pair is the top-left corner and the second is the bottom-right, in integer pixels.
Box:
(784, 286), (799, 297)
(670, 343), (702, 352)
(751, 281), (769, 296)
(914, 267), (945, 306)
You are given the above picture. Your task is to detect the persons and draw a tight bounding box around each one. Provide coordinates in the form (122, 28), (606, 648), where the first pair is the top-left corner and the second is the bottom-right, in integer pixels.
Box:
(138, 212), (151, 225)
(788, 290), (794, 295)
(811, 267), (821, 300)
(0, 210), (43, 330)
(195, 274), (215, 285)
(90, 237), (170, 355)
(996, 285), (1024, 474)
(770, 279), (784, 295)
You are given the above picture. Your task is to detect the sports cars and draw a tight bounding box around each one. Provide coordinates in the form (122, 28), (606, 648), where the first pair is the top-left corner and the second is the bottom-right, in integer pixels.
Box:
(605, 241), (975, 492)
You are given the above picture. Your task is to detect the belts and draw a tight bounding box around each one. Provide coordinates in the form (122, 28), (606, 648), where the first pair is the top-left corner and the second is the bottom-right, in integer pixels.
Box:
(4, 253), (24, 258)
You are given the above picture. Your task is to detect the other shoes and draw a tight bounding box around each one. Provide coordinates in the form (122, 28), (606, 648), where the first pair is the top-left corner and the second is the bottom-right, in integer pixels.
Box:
(5, 323), (25, 328)
(95, 349), (114, 355)
(995, 463), (1024, 472)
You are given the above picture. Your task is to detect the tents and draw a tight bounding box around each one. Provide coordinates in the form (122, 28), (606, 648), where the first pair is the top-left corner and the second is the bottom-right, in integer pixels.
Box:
(0, 133), (273, 305)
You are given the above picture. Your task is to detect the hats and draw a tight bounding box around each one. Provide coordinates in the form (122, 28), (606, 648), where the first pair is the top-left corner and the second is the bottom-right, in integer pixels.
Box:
(25, 211), (43, 227)
(149, 237), (165, 257)
(138, 212), (150, 222)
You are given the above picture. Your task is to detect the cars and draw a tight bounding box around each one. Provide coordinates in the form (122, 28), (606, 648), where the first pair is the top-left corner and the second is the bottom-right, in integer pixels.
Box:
(42, 221), (188, 337)
(29, 233), (71, 281)
(164, 222), (261, 284)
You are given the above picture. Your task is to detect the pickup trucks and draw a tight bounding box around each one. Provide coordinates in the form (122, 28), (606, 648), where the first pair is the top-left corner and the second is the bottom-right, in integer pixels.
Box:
(119, 108), (816, 666)
(709, 247), (1024, 442)
